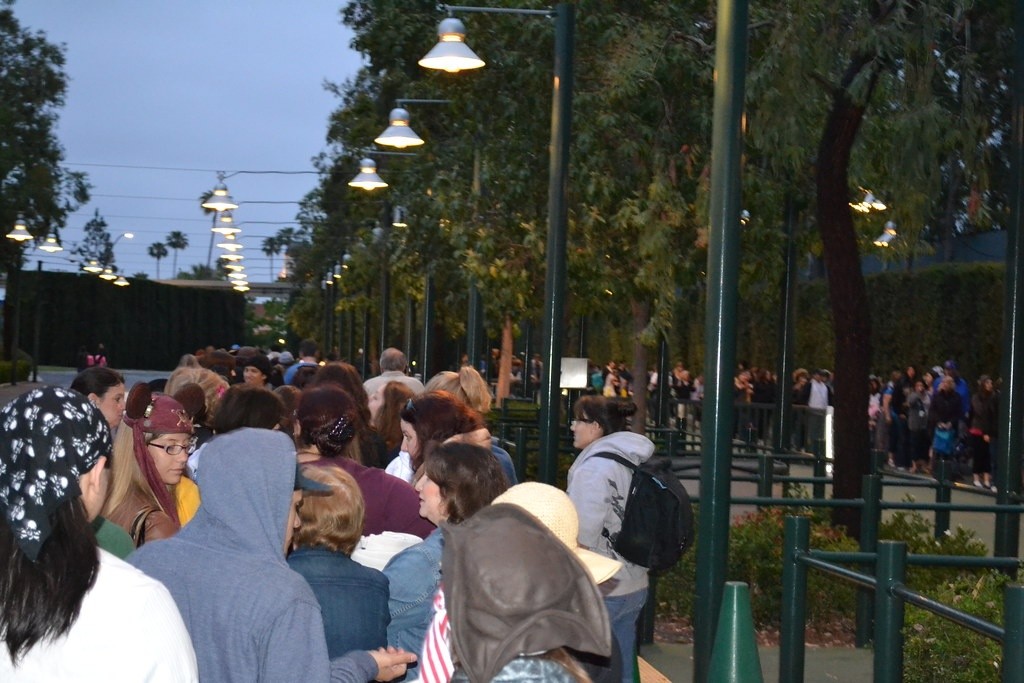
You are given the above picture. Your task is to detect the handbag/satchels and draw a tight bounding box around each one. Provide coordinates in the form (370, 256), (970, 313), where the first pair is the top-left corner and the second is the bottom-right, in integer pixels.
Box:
(932, 426), (955, 454)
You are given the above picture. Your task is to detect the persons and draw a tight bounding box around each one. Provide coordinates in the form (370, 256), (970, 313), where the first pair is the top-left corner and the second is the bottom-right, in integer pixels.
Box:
(125, 425), (417, 683)
(567, 394), (651, 683)
(66, 335), (624, 683)
(620, 347), (1005, 490)
(0, 385), (199, 683)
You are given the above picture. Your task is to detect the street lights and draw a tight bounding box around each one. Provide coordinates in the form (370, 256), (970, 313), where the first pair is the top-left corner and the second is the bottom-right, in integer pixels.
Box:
(322, 98), (488, 379)
(416, 0), (579, 486)
(775, 189), (901, 449)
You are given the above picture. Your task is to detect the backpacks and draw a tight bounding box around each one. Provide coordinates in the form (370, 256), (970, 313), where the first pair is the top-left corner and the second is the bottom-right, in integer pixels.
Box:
(584, 451), (694, 571)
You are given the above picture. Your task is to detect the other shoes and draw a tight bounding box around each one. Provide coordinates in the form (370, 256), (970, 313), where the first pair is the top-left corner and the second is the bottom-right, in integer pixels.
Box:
(983, 480), (992, 490)
(973, 480), (983, 488)
(887, 460), (895, 469)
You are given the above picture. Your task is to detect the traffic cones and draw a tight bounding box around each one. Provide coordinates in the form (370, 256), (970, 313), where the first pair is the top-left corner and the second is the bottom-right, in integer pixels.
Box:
(705, 580), (767, 683)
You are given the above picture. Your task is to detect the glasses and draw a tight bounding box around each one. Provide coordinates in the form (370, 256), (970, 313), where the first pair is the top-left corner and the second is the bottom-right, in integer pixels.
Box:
(570, 416), (595, 426)
(404, 397), (419, 412)
(146, 441), (195, 456)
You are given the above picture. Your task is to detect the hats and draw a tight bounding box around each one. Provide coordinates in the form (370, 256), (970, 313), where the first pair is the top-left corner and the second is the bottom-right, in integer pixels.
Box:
(440, 502), (611, 682)
(236, 355), (274, 376)
(229, 344), (240, 354)
(943, 359), (957, 369)
(868, 374), (883, 385)
(791, 367), (810, 383)
(278, 351), (294, 364)
(490, 481), (624, 586)
(294, 460), (333, 494)
(173, 383), (205, 417)
(976, 374), (992, 387)
